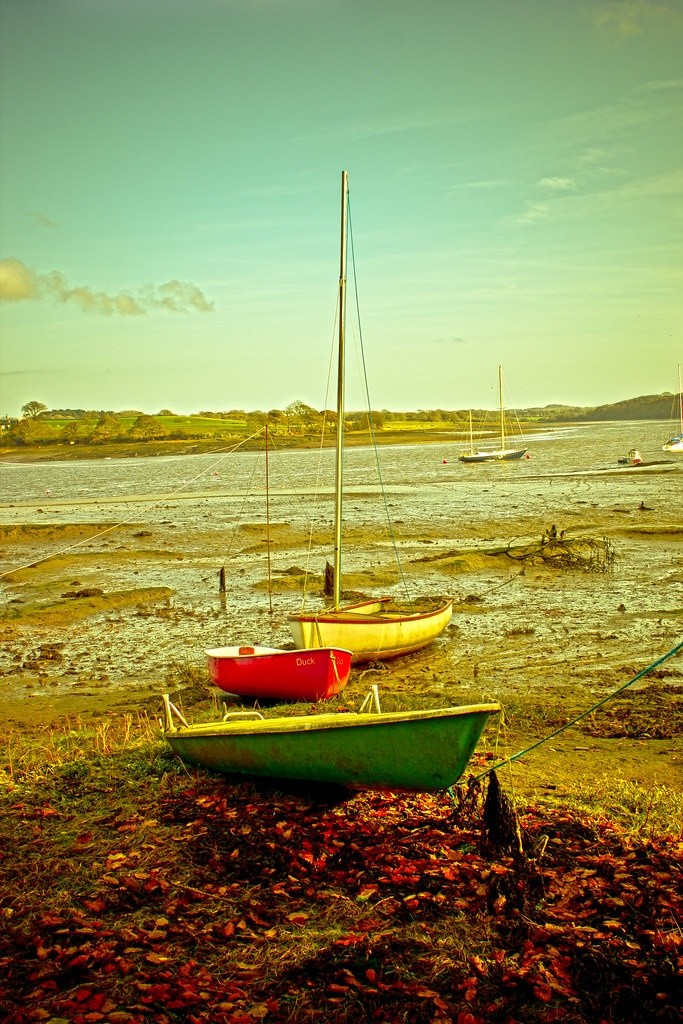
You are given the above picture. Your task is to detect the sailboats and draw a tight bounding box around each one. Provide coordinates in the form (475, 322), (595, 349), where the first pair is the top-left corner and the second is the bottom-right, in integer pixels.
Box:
(458, 365), (528, 461)
(661, 364), (683, 453)
(288, 169), (456, 664)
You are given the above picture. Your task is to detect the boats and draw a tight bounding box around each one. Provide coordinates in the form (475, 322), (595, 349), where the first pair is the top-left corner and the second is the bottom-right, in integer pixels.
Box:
(158, 684), (501, 788)
(618, 448), (642, 464)
(209, 639), (356, 701)
